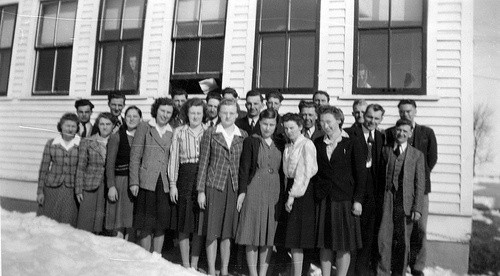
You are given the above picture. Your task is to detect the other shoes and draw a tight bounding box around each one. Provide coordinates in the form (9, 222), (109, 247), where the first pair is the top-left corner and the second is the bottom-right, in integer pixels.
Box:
(409, 263), (424, 276)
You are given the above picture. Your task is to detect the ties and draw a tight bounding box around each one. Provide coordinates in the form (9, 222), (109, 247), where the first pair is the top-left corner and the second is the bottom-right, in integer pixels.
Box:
(251, 119), (255, 128)
(393, 144), (401, 156)
(304, 129), (309, 137)
(209, 121), (214, 127)
(367, 131), (374, 144)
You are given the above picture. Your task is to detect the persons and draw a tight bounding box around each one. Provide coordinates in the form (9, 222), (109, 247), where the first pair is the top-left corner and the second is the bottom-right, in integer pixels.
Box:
(121, 55), (138, 91)
(75, 112), (117, 234)
(105, 105), (142, 241)
(129, 87), (438, 276)
(36, 113), (82, 228)
(358, 64), (371, 88)
(75, 99), (94, 138)
(91, 92), (125, 135)
